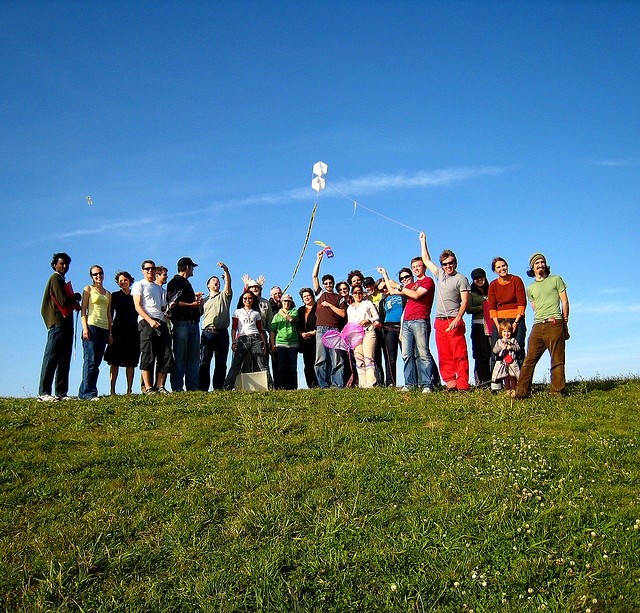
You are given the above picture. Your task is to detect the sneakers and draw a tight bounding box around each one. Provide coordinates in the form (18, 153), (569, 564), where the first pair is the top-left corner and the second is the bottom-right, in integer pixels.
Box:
(448, 387), (457, 392)
(505, 389), (511, 397)
(560, 388), (570, 398)
(146, 387), (158, 394)
(36, 394), (58, 403)
(401, 387), (409, 392)
(77, 396), (80, 400)
(422, 387), (432, 393)
(155, 386), (170, 394)
(91, 397), (100, 402)
(514, 394), (525, 400)
(510, 389), (516, 397)
(54, 395), (72, 401)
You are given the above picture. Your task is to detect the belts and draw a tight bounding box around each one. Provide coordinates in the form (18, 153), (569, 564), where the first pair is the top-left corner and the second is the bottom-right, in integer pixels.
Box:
(438, 317), (448, 320)
(541, 318), (555, 324)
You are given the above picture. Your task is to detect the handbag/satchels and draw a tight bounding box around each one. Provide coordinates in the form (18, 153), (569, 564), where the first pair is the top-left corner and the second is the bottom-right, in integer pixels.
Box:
(49, 273), (80, 319)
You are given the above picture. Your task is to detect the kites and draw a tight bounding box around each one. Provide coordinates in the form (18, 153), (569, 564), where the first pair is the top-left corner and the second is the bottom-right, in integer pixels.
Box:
(322, 322), (364, 386)
(86, 195), (93, 205)
(312, 175), (325, 192)
(312, 160), (327, 176)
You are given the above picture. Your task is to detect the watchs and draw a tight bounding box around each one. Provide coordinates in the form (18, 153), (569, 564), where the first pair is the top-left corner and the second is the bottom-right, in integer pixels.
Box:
(398, 284), (403, 291)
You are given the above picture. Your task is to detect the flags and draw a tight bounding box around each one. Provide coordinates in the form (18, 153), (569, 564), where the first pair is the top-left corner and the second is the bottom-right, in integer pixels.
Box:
(314, 239), (334, 257)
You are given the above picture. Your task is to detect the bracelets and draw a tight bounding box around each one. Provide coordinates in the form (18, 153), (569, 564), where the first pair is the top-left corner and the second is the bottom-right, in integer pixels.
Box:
(232, 342), (237, 344)
(514, 321), (518, 323)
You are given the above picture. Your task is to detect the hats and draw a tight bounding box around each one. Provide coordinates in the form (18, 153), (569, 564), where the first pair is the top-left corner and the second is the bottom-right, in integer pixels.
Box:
(362, 277), (375, 286)
(177, 257), (199, 267)
(280, 294), (293, 301)
(322, 274), (334, 281)
(471, 268), (487, 280)
(247, 280), (262, 290)
(528, 252), (547, 269)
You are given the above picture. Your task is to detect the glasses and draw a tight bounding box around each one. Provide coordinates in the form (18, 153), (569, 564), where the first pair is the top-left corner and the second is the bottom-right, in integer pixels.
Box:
(378, 284), (387, 293)
(399, 274), (412, 280)
(324, 281), (333, 285)
(441, 260), (453, 265)
(142, 266), (156, 270)
(90, 272), (105, 276)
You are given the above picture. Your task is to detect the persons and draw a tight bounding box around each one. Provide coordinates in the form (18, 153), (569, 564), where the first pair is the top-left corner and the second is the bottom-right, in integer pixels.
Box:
(37, 252), (82, 402)
(221, 290), (275, 390)
(104, 270), (140, 397)
(515, 252), (570, 398)
(297, 287), (318, 388)
(270, 294), (298, 389)
(78, 264), (113, 401)
(492, 321), (521, 397)
(312, 250), (347, 389)
(198, 261), (233, 391)
(488, 256), (527, 394)
(377, 256), (435, 393)
(269, 285), (296, 315)
(466, 267), (491, 386)
(131, 259), (176, 394)
(242, 274), (275, 390)
(419, 231), (471, 392)
(337, 267), (441, 391)
(167, 256), (204, 391)
(347, 285), (380, 388)
